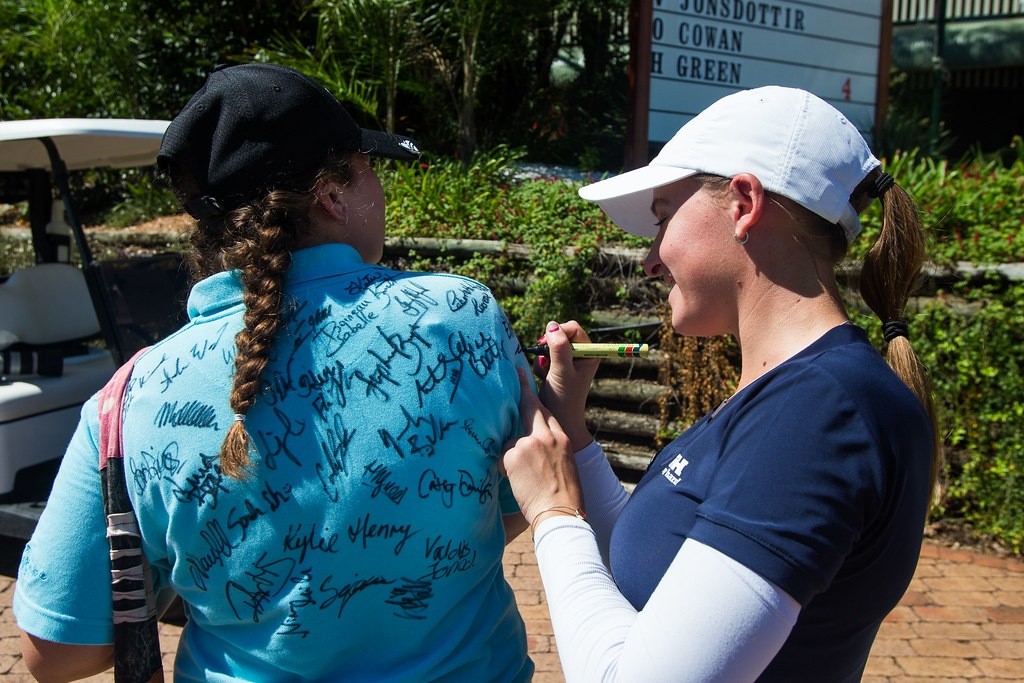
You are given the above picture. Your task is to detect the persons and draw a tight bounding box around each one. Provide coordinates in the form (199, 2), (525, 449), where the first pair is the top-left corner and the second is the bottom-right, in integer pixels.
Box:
(13, 64), (538, 683)
(498, 86), (938, 683)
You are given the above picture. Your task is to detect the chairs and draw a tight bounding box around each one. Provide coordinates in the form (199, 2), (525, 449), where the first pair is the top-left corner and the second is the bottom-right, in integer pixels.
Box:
(0, 264), (101, 495)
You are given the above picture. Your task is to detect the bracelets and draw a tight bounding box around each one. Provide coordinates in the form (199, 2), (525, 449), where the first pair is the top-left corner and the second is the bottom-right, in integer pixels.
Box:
(531, 506), (587, 542)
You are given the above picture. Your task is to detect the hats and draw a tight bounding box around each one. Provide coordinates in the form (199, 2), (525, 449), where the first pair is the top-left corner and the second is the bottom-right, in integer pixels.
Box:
(578, 86), (886, 247)
(157, 64), (423, 219)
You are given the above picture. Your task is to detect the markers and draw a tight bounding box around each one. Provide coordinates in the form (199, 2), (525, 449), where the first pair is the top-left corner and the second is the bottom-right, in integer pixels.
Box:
(522, 342), (650, 359)
